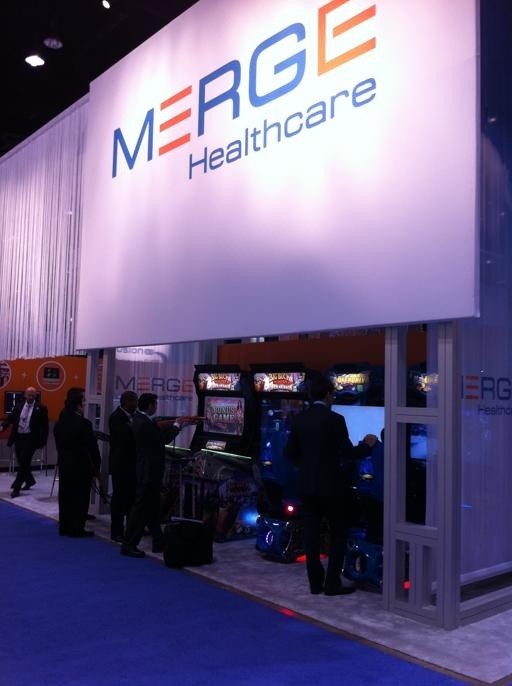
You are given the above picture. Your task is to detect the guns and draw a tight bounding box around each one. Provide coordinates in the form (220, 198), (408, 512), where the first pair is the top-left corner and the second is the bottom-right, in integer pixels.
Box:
(153, 416), (207, 430)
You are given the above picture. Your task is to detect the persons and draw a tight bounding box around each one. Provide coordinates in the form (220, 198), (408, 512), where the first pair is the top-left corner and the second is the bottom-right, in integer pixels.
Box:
(119, 393), (184, 557)
(54, 398), (105, 538)
(0, 386), (49, 497)
(286, 376), (379, 596)
(108, 390), (201, 543)
(66, 387), (87, 417)
(380, 429), (384, 446)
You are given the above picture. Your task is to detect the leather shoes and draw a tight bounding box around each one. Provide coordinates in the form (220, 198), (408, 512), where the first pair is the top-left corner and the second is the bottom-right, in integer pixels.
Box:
(111, 535), (125, 542)
(11, 487), (19, 498)
(73, 530), (94, 537)
(120, 543), (145, 558)
(325, 584), (356, 596)
(87, 514), (96, 520)
(22, 484), (30, 490)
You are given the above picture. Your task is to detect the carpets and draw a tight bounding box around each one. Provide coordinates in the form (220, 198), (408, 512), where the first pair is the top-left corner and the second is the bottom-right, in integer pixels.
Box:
(1, 496), (490, 686)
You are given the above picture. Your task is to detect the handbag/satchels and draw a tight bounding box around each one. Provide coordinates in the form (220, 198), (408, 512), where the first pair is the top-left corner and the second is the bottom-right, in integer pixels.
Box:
(161, 520), (214, 567)
(256, 518), (295, 558)
